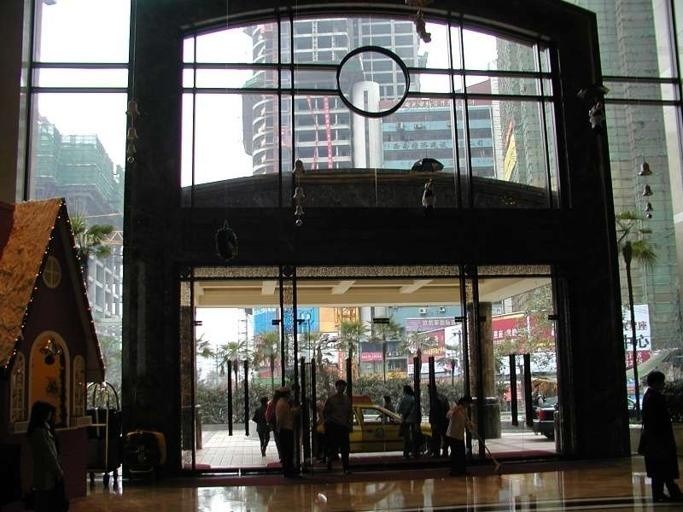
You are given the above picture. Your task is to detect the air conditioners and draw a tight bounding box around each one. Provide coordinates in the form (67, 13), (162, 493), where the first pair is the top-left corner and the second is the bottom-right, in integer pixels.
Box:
(396, 121), (423, 131)
(418, 305), (446, 315)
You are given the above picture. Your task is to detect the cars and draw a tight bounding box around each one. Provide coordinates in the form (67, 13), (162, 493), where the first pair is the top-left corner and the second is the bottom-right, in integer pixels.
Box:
(532, 395), (644, 440)
(349, 395), (446, 458)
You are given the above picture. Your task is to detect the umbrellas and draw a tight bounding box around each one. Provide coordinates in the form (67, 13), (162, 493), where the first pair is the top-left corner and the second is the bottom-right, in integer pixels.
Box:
(410, 156), (444, 176)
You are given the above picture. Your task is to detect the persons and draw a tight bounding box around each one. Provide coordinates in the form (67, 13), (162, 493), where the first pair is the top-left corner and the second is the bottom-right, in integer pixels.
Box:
(531, 382), (546, 435)
(252, 396), (269, 456)
(274, 387), (290, 470)
(49, 404), (59, 454)
(445, 393), (473, 475)
(639, 370), (682, 502)
(264, 388), (283, 463)
(287, 383), (302, 467)
(374, 394), (395, 423)
(25, 400), (67, 511)
(396, 385), (417, 457)
(322, 379), (353, 476)
(426, 383), (449, 457)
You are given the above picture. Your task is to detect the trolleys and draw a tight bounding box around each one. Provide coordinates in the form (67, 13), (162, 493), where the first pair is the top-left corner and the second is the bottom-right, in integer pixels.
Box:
(82, 381), (124, 486)
